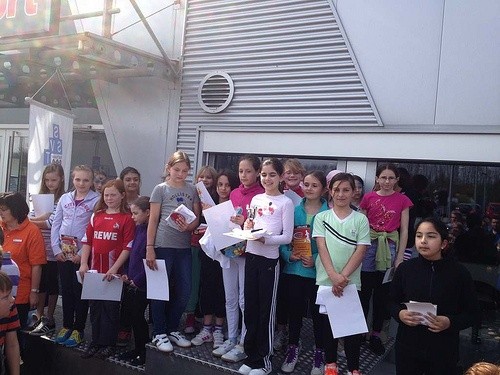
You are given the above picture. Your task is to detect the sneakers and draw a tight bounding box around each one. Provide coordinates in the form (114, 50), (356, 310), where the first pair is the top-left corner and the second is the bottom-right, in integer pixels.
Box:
(347, 368), (362, 375)
(370, 335), (385, 354)
(185, 315), (195, 333)
(191, 329), (213, 345)
(167, 330), (192, 347)
(118, 325), (131, 346)
(310, 350), (325, 375)
(212, 339), (239, 357)
(281, 346), (299, 373)
(324, 362), (338, 375)
(151, 333), (174, 352)
(237, 361), (272, 375)
(213, 330), (224, 348)
(220, 344), (246, 362)
(274, 330), (285, 350)
(24, 315), (116, 359)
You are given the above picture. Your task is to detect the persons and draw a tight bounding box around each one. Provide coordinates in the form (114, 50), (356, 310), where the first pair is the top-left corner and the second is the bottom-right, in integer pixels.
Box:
(360, 164), (413, 355)
(387, 217), (480, 375)
(238, 158), (294, 375)
(28, 158), (412, 375)
(0, 191), (48, 375)
(446, 212), (500, 265)
(147, 150), (200, 352)
(312, 174), (372, 375)
(466, 362), (500, 375)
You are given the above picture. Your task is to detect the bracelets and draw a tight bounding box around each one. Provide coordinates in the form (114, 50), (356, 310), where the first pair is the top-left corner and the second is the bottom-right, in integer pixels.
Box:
(147, 245), (154, 246)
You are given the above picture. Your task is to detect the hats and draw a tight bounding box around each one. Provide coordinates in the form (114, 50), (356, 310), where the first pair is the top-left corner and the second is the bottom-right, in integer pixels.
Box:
(325, 170), (344, 183)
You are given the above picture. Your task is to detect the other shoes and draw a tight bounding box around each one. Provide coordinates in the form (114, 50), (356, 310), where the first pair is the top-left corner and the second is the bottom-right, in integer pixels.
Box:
(337, 340), (346, 357)
(118, 349), (146, 364)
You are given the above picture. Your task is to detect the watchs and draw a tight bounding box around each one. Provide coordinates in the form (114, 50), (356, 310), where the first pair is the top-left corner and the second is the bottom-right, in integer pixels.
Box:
(31, 289), (39, 293)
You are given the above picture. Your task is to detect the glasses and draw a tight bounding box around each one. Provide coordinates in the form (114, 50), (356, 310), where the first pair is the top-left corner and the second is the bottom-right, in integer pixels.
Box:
(377, 176), (395, 182)
